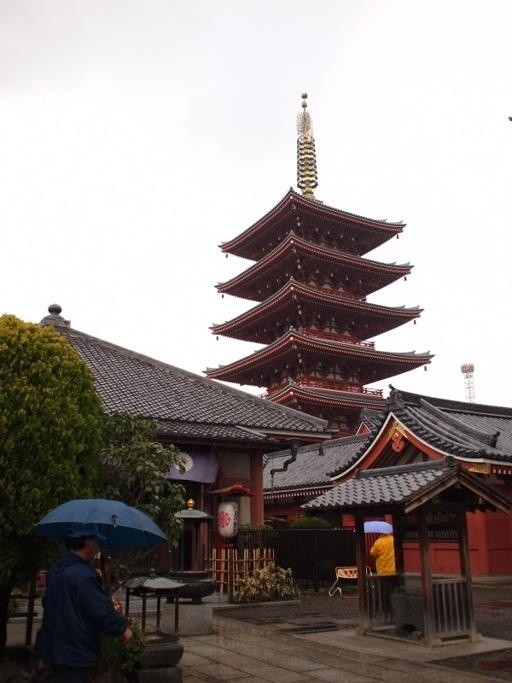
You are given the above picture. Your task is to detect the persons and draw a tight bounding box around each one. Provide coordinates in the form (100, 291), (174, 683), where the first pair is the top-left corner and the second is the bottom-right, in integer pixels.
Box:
(367, 534), (397, 577)
(34, 525), (134, 683)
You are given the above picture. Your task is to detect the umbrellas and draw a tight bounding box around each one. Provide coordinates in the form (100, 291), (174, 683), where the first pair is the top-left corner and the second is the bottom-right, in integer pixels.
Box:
(351, 518), (394, 534)
(30, 495), (168, 554)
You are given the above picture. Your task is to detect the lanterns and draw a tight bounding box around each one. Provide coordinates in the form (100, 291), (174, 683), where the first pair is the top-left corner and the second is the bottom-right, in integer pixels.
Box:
(214, 497), (240, 546)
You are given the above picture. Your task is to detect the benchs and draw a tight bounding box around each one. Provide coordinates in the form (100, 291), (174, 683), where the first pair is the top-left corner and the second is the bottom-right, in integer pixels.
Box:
(329, 564), (372, 597)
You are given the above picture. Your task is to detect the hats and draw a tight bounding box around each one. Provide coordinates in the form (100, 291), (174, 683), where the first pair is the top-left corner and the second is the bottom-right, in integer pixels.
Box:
(65, 523), (108, 541)
